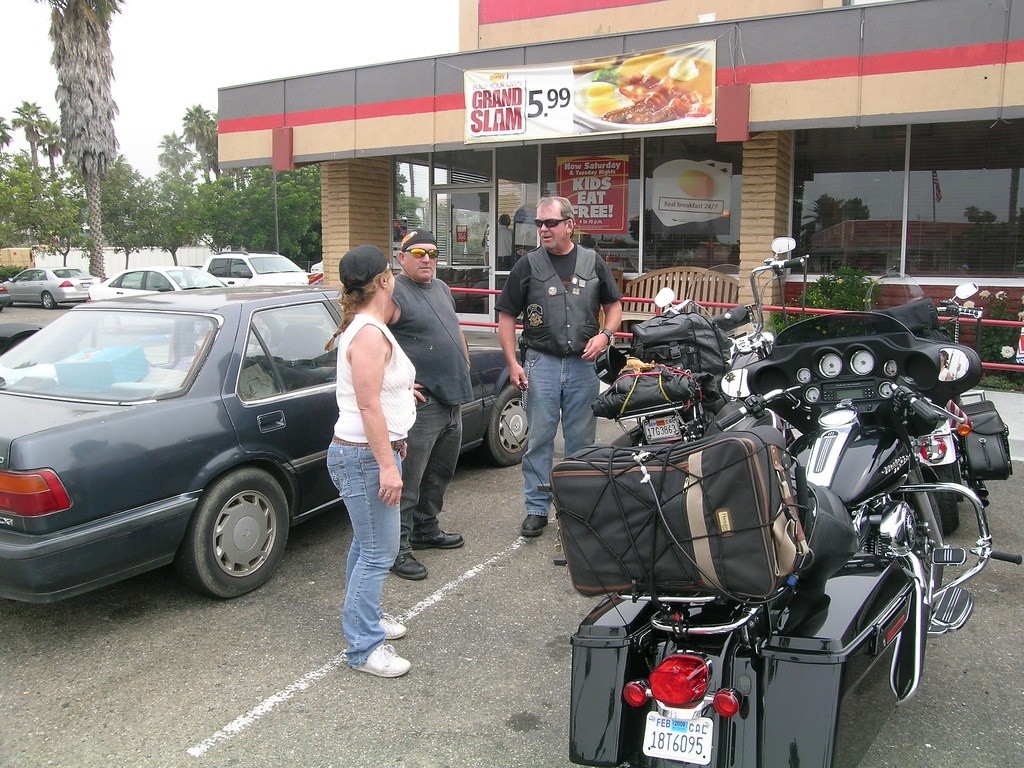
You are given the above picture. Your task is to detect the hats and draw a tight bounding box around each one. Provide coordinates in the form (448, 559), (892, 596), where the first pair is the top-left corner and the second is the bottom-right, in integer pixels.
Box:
(400, 228), (437, 251)
(338, 244), (388, 302)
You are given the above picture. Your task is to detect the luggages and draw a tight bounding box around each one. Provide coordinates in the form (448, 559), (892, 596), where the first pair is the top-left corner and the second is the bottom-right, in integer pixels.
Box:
(550, 425), (809, 599)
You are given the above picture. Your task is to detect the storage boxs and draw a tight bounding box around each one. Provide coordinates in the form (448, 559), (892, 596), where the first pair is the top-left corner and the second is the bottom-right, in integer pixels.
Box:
(568, 552), (917, 768)
(54, 345), (149, 390)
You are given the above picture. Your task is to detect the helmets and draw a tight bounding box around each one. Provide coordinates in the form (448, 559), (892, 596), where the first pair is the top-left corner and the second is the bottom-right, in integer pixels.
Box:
(593, 346), (629, 385)
(790, 593), (831, 639)
(799, 478), (856, 585)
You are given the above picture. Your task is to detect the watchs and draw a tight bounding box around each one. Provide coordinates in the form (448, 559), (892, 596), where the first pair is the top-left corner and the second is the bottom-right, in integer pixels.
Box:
(599, 329), (615, 346)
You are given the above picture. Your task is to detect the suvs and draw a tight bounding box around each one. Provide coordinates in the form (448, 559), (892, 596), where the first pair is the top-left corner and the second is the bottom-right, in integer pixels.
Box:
(202, 250), (309, 286)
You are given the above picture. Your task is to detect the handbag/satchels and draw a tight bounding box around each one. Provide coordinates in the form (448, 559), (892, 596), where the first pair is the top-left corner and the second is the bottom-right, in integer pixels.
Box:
(629, 311), (731, 401)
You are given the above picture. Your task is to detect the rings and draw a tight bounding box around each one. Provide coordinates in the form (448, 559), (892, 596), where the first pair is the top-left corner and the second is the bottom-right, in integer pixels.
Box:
(385, 491), (391, 497)
(380, 488), (385, 494)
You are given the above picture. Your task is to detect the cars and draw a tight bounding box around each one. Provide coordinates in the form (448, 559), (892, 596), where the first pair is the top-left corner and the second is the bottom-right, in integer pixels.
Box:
(307, 260), (323, 286)
(0, 267), (101, 308)
(0, 285), (530, 600)
(88, 265), (227, 300)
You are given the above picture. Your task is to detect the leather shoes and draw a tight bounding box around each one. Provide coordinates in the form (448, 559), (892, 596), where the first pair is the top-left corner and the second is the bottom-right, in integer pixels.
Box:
(390, 552), (428, 580)
(410, 529), (465, 550)
(521, 514), (548, 536)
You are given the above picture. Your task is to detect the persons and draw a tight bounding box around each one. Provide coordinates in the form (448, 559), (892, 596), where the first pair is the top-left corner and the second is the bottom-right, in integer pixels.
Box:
(498, 213), (514, 271)
(671, 248), (695, 266)
(325, 244), (417, 677)
(938, 350), (952, 381)
(494, 196), (623, 538)
(385, 229), (474, 580)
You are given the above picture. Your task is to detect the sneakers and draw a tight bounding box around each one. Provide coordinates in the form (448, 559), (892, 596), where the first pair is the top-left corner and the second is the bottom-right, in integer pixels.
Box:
(378, 611), (406, 639)
(352, 644), (412, 677)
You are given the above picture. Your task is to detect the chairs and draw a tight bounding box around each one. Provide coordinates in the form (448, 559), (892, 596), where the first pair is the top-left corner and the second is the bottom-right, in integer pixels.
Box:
(269, 324), (336, 392)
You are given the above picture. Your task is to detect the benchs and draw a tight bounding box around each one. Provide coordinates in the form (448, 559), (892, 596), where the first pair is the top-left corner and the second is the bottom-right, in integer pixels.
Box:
(619, 267), (739, 344)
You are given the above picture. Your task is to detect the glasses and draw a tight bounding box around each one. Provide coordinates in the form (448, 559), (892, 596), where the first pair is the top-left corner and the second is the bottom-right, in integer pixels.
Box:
(387, 263), (394, 272)
(404, 248), (438, 258)
(533, 217), (569, 228)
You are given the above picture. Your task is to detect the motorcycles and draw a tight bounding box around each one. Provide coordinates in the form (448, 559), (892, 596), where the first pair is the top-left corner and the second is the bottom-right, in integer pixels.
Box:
(533, 281), (1011, 768)
(589, 235), (809, 449)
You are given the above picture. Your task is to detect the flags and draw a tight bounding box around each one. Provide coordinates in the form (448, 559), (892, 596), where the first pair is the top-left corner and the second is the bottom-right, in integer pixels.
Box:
(932, 170), (942, 203)
